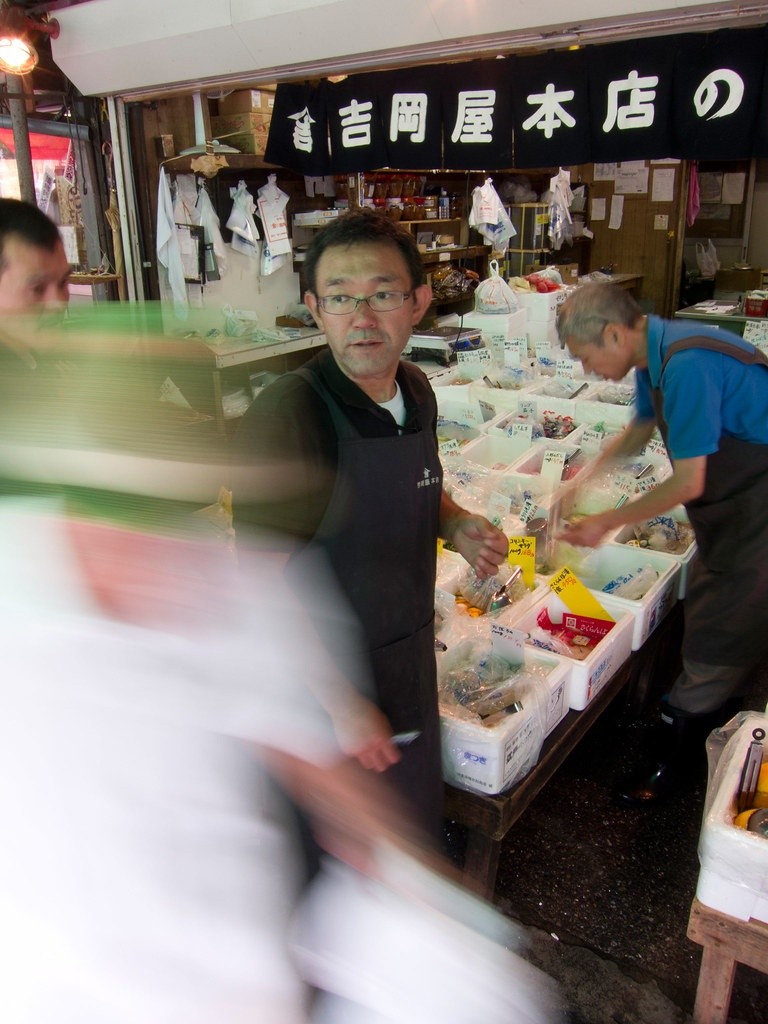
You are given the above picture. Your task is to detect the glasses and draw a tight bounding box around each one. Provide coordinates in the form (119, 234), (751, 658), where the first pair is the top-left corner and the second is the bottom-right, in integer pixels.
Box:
(315, 289), (415, 316)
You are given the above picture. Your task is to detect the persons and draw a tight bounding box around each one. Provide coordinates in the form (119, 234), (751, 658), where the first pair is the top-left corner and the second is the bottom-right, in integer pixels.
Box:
(0, 501), (457, 1024)
(0, 197), (70, 380)
(237, 209), (510, 898)
(547, 283), (768, 809)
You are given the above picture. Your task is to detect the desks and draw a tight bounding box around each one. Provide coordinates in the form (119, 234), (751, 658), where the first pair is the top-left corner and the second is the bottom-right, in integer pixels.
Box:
(671, 300), (768, 334)
(197, 325), (327, 446)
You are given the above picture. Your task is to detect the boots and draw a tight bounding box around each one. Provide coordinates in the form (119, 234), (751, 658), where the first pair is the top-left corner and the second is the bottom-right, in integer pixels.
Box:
(615, 705), (704, 810)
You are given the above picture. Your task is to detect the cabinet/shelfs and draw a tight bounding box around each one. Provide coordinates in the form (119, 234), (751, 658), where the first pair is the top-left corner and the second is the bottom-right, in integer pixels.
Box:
(290, 173), (491, 329)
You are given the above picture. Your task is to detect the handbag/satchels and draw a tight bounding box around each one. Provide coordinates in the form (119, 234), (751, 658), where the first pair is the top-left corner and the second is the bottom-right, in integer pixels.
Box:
(474, 259), (517, 314)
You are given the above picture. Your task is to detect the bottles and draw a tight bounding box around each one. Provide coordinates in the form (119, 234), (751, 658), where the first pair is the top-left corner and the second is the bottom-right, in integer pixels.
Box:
(438, 191), (449, 219)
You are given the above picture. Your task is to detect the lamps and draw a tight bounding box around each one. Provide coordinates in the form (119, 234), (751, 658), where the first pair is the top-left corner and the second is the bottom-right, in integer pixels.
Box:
(0, 8), (59, 75)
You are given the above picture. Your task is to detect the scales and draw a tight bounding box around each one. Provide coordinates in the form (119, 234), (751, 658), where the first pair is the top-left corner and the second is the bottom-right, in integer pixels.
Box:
(412, 325), (481, 365)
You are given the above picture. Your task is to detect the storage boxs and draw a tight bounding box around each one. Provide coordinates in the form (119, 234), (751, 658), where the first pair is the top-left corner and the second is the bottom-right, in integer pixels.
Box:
(412, 285), (682, 797)
(697, 718), (768, 923)
(744, 297), (768, 318)
